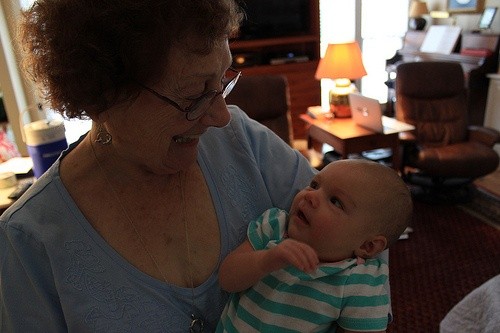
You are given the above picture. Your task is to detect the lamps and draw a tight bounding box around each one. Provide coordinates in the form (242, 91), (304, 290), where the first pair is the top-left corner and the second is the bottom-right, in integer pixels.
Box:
(315, 40), (367, 118)
(408, 1), (430, 31)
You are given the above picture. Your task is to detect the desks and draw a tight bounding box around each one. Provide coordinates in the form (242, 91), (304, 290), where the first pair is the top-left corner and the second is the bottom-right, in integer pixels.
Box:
(297, 111), (415, 173)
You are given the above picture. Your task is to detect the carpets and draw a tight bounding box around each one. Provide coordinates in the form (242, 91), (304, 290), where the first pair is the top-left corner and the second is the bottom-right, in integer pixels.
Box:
(384, 188), (500, 333)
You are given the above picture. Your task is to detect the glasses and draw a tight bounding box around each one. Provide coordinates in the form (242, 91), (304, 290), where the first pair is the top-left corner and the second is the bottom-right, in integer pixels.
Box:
(128, 67), (243, 122)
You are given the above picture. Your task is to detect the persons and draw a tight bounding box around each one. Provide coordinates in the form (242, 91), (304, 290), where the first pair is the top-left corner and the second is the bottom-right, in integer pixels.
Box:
(216, 159), (412, 333)
(0, 0), (393, 333)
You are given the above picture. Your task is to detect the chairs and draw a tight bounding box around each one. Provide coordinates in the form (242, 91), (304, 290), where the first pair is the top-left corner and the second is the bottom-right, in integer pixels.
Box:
(394, 61), (500, 207)
(224, 76), (295, 148)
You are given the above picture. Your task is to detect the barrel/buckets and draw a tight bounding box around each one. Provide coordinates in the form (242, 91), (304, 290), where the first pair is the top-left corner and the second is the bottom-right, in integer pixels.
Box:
(20, 102), (68, 179)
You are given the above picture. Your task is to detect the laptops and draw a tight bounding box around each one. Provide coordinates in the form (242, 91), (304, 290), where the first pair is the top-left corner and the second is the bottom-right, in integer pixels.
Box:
(348, 93), (415, 134)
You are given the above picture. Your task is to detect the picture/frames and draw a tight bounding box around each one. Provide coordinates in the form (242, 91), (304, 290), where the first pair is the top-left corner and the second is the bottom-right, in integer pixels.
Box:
(446, 0), (485, 15)
(477, 6), (498, 29)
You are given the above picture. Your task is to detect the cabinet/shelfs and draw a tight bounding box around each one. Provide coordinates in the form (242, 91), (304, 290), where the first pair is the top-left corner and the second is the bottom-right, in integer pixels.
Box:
(223, 0), (322, 141)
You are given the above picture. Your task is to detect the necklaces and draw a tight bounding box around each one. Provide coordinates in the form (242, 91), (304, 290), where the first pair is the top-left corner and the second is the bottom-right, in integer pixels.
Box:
(90, 133), (204, 333)
